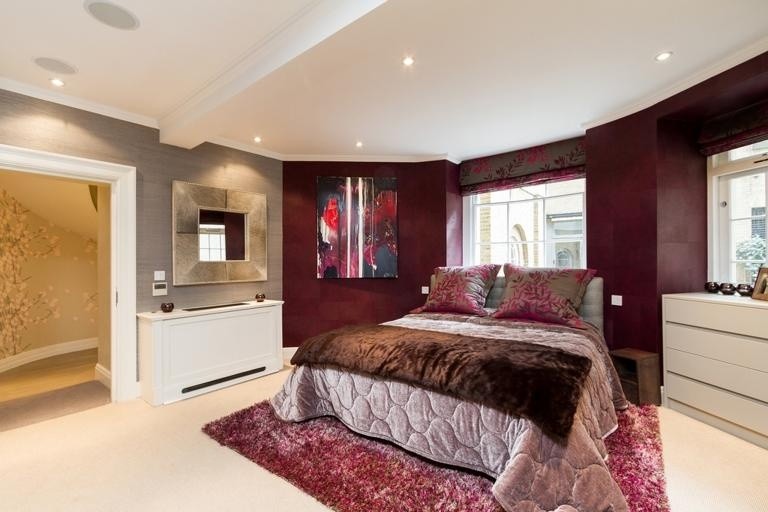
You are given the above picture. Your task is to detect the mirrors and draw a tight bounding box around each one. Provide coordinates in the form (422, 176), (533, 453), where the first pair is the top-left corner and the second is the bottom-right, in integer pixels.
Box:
(197, 205), (250, 264)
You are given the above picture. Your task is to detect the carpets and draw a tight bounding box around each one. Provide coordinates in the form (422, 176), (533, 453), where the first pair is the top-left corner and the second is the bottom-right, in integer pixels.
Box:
(202, 400), (670, 512)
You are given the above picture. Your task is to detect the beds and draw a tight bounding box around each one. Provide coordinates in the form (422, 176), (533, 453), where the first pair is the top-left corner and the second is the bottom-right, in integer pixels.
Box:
(270, 276), (628, 512)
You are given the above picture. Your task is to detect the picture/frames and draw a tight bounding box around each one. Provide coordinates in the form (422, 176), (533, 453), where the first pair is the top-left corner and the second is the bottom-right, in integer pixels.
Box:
(752, 268), (768, 301)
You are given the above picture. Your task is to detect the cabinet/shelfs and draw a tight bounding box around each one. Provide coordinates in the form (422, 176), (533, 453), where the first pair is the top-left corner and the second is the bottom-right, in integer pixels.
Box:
(136, 300), (285, 405)
(661, 292), (768, 449)
(608, 348), (660, 407)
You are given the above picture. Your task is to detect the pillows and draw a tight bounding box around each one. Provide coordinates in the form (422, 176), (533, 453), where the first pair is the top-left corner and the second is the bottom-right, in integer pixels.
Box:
(489, 264), (598, 330)
(409, 265), (501, 316)
